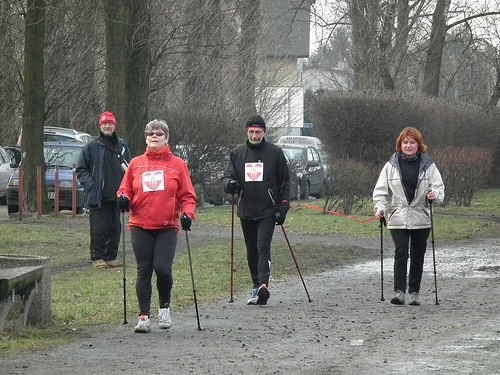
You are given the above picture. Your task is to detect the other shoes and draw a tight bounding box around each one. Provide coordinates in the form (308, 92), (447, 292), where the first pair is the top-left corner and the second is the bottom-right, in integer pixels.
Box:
(92, 260), (109, 268)
(106, 260), (123, 266)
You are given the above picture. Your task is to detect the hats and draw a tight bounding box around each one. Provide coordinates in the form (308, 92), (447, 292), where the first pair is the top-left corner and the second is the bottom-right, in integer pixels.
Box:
(99, 112), (116, 127)
(246, 115), (266, 132)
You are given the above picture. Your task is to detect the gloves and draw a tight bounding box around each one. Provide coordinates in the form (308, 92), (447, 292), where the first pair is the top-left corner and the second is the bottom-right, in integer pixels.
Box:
(180, 217), (191, 230)
(227, 179), (241, 195)
(117, 197), (130, 213)
(273, 202), (290, 225)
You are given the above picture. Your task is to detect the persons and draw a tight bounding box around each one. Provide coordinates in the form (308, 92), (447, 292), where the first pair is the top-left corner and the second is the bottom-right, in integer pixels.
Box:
(76, 111), (131, 268)
(115, 119), (195, 332)
(222, 116), (291, 304)
(373, 127), (445, 304)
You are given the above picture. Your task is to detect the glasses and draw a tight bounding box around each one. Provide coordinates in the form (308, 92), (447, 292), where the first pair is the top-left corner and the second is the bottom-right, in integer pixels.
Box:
(147, 132), (167, 137)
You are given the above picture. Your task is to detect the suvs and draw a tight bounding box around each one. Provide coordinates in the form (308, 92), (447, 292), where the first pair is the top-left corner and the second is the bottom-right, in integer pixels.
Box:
(43, 125), (91, 144)
(174, 145), (232, 182)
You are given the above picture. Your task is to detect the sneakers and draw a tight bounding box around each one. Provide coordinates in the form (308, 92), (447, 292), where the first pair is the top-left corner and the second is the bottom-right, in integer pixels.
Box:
(247, 289), (259, 305)
(409, 292), (421, 305)
(158, 306), (172, 328)
(391, 290), (406, 305)
(135, 316), (151, 333)
(257, 286), (270, 305)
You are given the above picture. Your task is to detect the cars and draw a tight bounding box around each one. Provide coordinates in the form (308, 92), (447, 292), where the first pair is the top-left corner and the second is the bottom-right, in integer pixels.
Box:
(223, 151), (301, 201)
(0, 145), (14, 205)
(6, 141), (87, 214)
(274, 142), (325, 199)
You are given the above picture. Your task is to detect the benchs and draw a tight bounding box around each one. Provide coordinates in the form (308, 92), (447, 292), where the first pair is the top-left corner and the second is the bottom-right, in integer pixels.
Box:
(0, 265), (44, 331)
